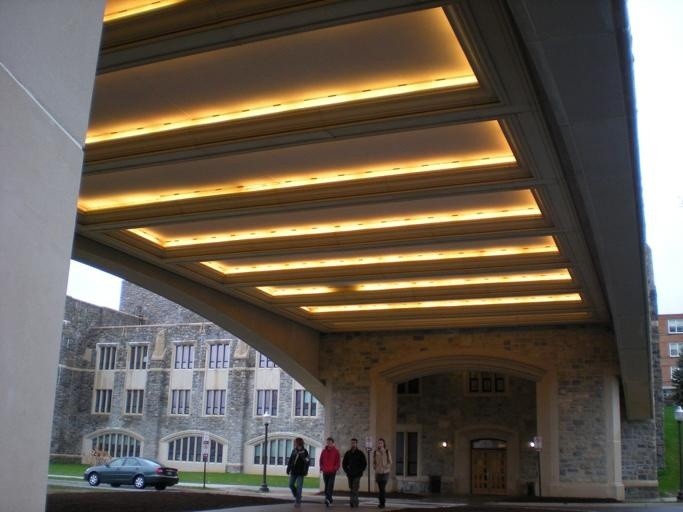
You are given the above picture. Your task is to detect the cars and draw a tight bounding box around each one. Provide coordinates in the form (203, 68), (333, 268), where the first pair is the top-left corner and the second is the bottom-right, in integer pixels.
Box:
(84, 456), (180, 491)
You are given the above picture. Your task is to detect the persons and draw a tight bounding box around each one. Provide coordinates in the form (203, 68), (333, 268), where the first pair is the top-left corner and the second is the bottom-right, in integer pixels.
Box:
(372, 438), (392, 509)
(342, 438), (367, 509)
(286, 438), (310, 507)
(319, 437), (341, 506)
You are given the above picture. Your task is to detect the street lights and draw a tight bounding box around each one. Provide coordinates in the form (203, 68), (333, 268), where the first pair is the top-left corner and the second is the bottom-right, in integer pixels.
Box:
(674, 404), (683, 504)
(258, 411), (271, 494)
